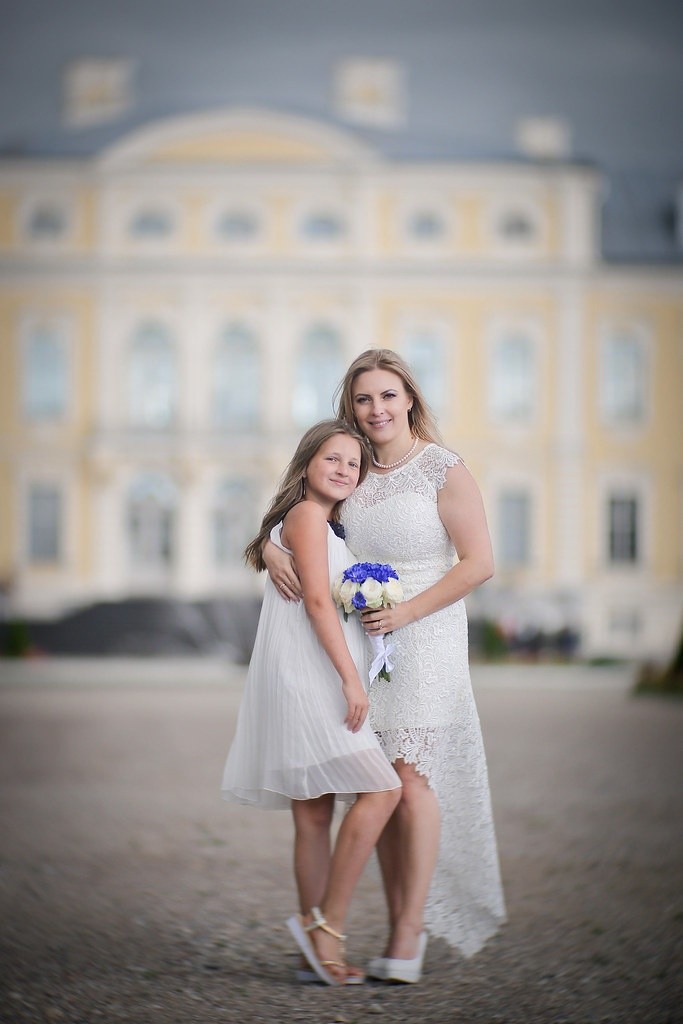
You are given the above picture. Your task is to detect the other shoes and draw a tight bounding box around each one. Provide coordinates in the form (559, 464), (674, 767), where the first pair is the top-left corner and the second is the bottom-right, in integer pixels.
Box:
(367, 957), (386, 979)
(382, 931), (427, 983)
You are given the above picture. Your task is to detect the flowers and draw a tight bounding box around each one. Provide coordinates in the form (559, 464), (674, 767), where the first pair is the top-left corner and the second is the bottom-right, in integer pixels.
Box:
(335, 561), (401, 681)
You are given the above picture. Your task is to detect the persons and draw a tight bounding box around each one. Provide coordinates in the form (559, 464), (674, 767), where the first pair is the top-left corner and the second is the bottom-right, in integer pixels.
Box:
(218, 419), (402, 985)
(261, 350), (507, 985)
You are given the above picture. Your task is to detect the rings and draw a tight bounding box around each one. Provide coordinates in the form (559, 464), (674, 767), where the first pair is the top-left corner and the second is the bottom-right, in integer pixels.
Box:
(377, 620), (383, 629)
(279, 584), (285, 589)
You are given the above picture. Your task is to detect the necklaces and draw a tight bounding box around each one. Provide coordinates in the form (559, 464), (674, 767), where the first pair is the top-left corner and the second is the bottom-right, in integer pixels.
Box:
(371, 436), (419, 469)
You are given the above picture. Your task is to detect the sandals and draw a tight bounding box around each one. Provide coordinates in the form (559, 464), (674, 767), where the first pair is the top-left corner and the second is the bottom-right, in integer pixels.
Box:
(286, 907), (347, 987)
(294, 960), (365, 984)
